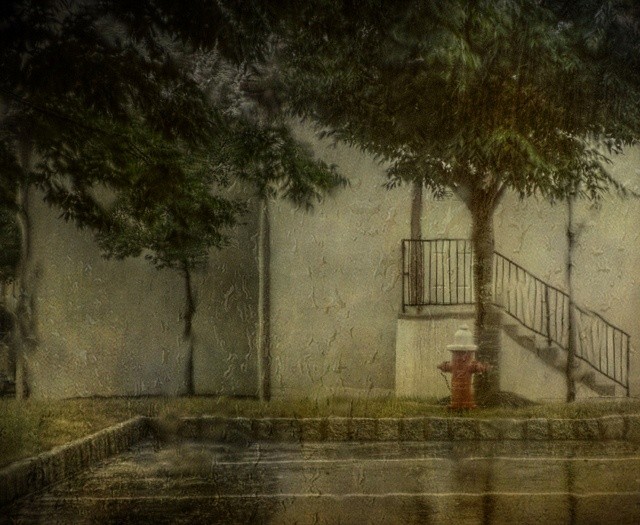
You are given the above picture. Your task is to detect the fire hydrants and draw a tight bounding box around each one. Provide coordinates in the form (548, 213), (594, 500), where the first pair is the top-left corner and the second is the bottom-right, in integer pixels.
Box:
(437, 324), (491, 410)
